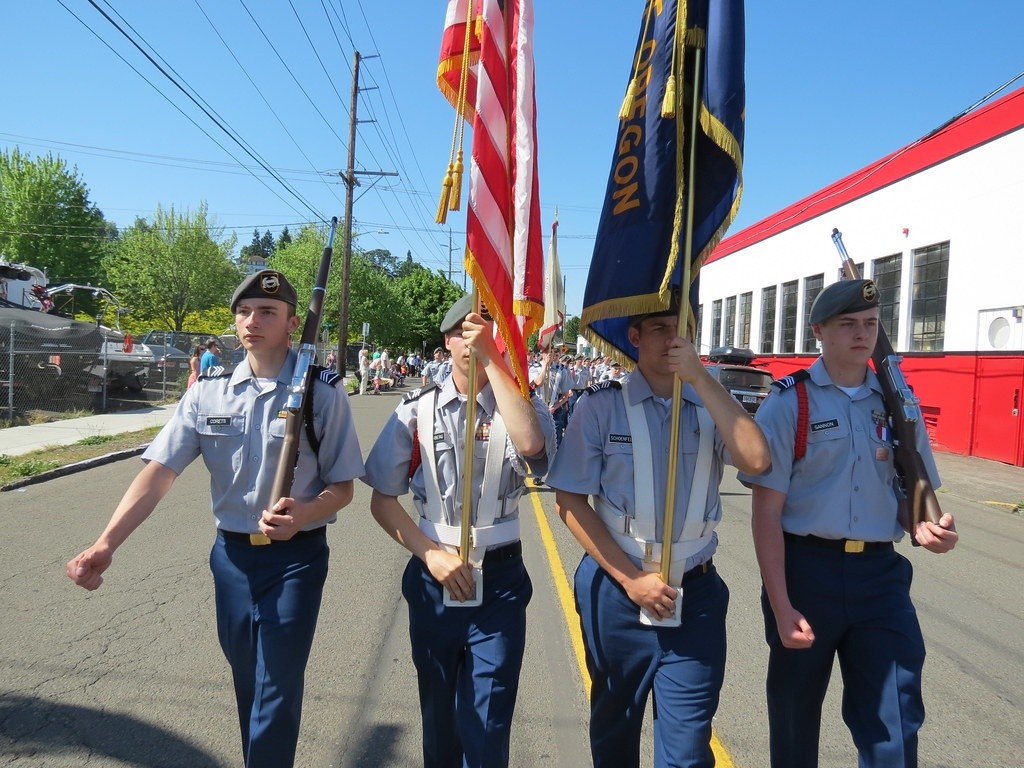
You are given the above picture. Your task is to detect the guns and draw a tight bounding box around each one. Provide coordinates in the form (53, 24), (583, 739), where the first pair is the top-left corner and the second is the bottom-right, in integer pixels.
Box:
(261, 214), (338, 527)
(829, 225), (949, 547)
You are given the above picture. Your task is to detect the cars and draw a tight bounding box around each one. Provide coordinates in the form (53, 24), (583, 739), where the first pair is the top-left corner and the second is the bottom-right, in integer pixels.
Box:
(138, 343), (191, 389)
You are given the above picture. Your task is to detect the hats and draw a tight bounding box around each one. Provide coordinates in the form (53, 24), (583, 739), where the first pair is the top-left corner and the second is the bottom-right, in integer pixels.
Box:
(629, 283), (681, 325)
(230, 269), (297, 314)
(527, 349), (543, 357)
(551, 345), (614, 363)
(440, 294), (494, 333)
(433, 347), (451, 357)
(808, 279), (881, 325)
(611, 363), (622, 368)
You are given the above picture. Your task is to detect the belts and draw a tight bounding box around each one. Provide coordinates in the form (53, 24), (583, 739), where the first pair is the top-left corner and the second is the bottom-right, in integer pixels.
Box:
(606, 526), (713, 563)
(419, 516), (521, 550)
(483, 540), (522, 561)
(217, 525), (326, 546)
(682, 558), (712, 584)
(783, 531), (893, 553)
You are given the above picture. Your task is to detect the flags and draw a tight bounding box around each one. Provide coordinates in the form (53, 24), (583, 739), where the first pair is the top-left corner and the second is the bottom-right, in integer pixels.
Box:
(435, 0), (545, 407)
(578, 0), (746, 375)
(537, 220), (565, 355)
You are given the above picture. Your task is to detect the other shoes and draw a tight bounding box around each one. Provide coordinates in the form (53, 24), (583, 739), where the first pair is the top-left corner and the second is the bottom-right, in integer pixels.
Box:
(533, 478), (544, 485)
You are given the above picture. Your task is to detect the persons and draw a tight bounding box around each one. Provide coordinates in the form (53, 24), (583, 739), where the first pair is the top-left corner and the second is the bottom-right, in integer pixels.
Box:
(736, 279), (959, 768)
(543, 283), (774, 768)
(358, 294), (559, 768)
(187, 339), (221, 390)
(64, 268), (367, 768)
(325, 349), (336, 369)
(357, 346), (628, 484)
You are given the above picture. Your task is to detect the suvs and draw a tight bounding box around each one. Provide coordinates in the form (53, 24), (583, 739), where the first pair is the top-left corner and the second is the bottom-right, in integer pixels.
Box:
(0, 263), (103, 401)
(46, 284), (155, 391)
(141, 330), (248, 366)
(702, 347), (778, 419)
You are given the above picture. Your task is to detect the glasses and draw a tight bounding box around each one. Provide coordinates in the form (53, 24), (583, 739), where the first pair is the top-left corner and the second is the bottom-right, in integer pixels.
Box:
(214, 345), (218, 347)
(201, 348), (205, 350)
